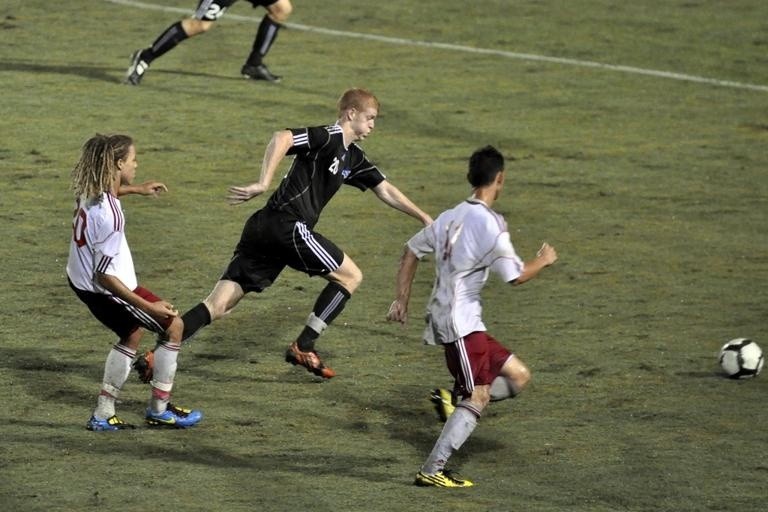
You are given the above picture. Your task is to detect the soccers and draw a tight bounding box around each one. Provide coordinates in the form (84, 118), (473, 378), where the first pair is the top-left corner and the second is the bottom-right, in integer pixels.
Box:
(718, 338), (764, 380)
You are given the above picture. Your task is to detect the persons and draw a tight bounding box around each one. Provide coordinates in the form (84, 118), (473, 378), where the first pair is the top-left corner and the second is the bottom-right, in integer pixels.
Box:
(126, 0), (293, 86)
(386, 144), (558, 490)
(133, 88), (434, 383)
(65, 131), (202, 431)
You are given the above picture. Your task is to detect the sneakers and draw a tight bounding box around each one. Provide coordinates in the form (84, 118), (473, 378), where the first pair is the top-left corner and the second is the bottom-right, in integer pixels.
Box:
(134, 351), (154, 383)
(127, 49), (150, 86)
(414, 467), (475, 492)
(430, 388), (456, 422)
(87, 415), (137, 431)
(240, 63), (283, 83)
(144, 402), (202, 428)
(284, 341), (336, 379)
